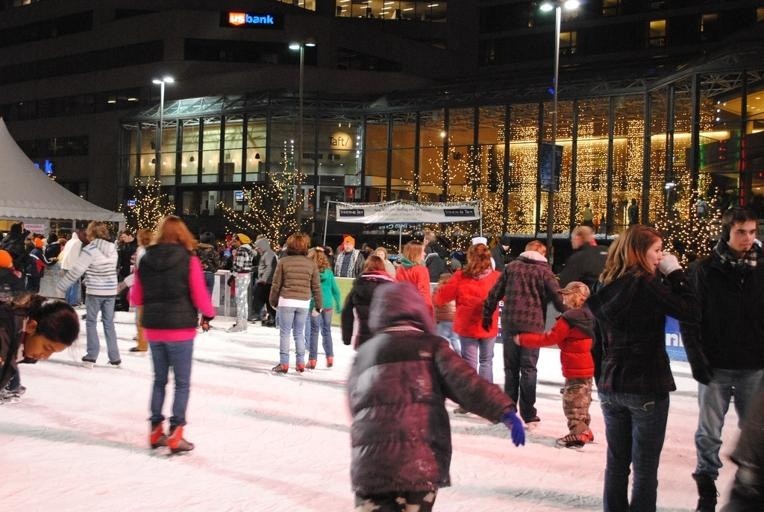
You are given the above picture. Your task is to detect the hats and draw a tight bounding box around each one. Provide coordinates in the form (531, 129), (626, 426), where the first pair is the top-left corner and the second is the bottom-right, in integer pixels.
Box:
(558, 281), (590, 299)
(236, 233), (252, 244)
(343, 236), (355, 245)
(471, 236), (487, 246)
(34, 238), (43, 248)
(0, 250), (12, 269)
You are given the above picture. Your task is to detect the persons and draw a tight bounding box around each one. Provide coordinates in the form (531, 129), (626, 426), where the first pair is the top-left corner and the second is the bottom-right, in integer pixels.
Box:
(483, 240), (570, 422)
(512, 281), (596, 447)
(557, 225), (601, 402)
(586, 224), (696, 512)
(679, 205), (764, 512)
(628, 199), (639, 223)
(583, 204), (594, 226)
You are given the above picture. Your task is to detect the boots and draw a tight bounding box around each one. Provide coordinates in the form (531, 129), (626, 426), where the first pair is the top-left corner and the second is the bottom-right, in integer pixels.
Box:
(149, 425), (167, 449)
(692, 473), (718, 511)
(168, 422), (194, 453)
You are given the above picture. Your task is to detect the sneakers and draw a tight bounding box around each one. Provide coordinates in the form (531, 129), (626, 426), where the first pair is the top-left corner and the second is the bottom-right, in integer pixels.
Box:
(556, 433), (585, 447)
(81, 356), (96, 363)
(129, 347), (146, 352)
(453, 405), (470, 417)
(521, 414), (541, 423)
(272, 357), (334, 374)
(584, 430), (595, 443)
(109, 357), (121, 365)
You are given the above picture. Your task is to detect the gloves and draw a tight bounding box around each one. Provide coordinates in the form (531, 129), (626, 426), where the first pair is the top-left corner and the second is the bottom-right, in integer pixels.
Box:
(500, 409), (526, 448)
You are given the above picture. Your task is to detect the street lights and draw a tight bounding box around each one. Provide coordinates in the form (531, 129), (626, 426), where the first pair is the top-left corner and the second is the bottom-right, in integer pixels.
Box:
(537, 1), (580, 269)
(288, 38), (315, 234)
(151, 75), (175, 210)
